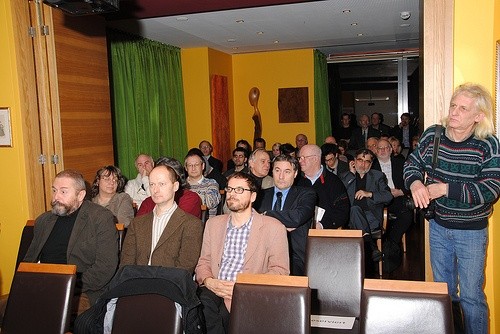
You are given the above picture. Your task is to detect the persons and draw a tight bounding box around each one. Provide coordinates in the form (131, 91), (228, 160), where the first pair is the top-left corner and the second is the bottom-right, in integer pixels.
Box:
(199, 112), (423, 279)
(119, 164), (205, 271)
(181, 153), (221, 219)
(88, 165), (135, 228)
(184, 148), (229, 215)
(0, 169), (119, 317)
(135, 157), (202, 221)
(184, 171), (291, 334)
(402, 83), (500, 334)
(124, 153), (155, 210)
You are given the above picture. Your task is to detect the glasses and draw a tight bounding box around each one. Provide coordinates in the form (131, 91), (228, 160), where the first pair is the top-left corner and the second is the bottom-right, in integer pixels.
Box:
(297, 155), (316, 161)
(225, 187), (253, 194)
(378, 146), (391, 150)
(325, 157), (335, 163)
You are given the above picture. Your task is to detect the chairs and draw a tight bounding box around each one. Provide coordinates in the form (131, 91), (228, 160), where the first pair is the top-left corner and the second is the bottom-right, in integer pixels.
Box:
(304, 228), (365, 334)
(359, 278), (456, 334)
(388, 192), (416, 254)
(0, 262), (77, 334)
(229, 272), (314, 334)
(101, 295), (184, 334)
(337, 205), (388, 276)
(15, 220), (125, 268)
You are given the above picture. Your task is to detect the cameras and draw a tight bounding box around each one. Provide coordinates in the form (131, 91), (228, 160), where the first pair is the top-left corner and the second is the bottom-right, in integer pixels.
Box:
(403, 194), (436, 220)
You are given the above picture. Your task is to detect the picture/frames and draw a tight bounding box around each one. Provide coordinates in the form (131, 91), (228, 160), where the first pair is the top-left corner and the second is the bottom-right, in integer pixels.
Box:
(0, 107), (13, 148)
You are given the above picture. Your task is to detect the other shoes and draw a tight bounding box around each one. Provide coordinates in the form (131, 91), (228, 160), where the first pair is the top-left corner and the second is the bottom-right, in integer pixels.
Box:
(366, 243), (382, 262)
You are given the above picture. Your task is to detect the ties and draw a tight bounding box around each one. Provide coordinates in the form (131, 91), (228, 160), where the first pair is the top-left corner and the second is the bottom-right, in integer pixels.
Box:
(363, 130), (365, 150)
(273, 192), (283, 211)
